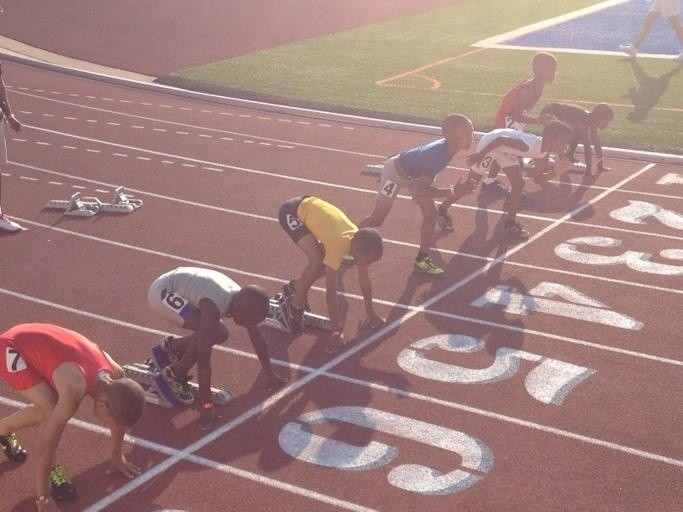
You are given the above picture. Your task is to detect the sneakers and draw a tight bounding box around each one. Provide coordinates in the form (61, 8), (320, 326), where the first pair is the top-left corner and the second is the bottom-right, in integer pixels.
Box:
(413, 256), (444, 275)
(0, 433), (26, 462)
(163, 366), (194, 405)
(48, 464), (75, 499)
(0, 213), (22, 231)
(483, 176), (507, 193)
(160, 337), (181, 366)
(284, 280), (312, 335)
(435, 203), (454, 230)
(503, 220), (529, 237)
(618, 43), (638, 56)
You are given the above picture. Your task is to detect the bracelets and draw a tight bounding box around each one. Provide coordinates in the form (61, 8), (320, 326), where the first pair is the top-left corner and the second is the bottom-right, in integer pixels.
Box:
(200, 402), (214, 411)
(34, 493), (53, 505)
(450, 186), (455, 198)
(329, 327), (343, 335)
(6, 112), (15, 119)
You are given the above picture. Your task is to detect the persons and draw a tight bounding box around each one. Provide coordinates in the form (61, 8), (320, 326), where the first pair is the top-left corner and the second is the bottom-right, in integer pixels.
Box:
(524, 102), (612, 186)
(618, 0), (683, 63)
(359, 113), (472, 275)
(0, 66), (21, 233)
(278, 195), (387, 355)
(147, 267), (288, 431)
(479, 51), (557, 194)
(1, 322), (146, 512)
(434, 120), (575, 238)
